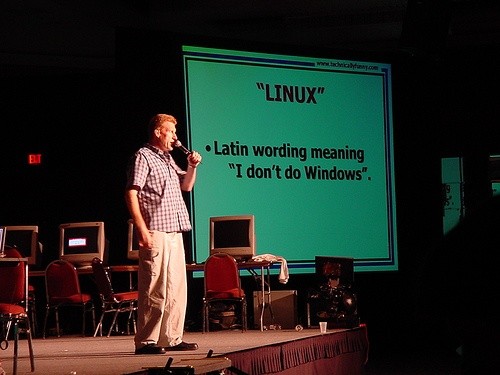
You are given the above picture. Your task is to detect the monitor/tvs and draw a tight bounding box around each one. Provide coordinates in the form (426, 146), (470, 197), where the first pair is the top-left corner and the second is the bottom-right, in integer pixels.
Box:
(59, 222), (109, 271)
(210, 215), (255, 260)
(127, 219), (139, 261)
(0, 225), (38, 265)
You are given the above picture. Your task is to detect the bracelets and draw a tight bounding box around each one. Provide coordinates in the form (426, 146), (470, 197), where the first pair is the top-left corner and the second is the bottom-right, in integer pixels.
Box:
(189, 164), (197, 168)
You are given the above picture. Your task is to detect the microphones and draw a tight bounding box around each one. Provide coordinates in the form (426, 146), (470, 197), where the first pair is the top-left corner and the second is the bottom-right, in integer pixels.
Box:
(174, 140), (192, 156)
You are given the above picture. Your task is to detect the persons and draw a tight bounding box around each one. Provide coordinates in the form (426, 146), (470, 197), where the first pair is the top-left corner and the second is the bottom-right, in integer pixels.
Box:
(125, 114), (201, 354)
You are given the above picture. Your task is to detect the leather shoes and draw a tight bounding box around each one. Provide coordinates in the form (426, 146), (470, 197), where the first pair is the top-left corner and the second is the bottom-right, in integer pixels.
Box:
(165, 341), (198, 351)
(135, 344), (166, 353)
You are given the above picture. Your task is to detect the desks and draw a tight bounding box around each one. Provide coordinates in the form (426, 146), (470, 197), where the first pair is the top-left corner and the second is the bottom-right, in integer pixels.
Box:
(27, 259), (274, 333)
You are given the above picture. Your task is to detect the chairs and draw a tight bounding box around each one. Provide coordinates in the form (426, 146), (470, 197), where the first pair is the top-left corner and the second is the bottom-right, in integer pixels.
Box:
(91, 258), (138, 338)
(0, 245), (35, 375)
(46, 258), (96, 338)
(203, 252), (247, 334)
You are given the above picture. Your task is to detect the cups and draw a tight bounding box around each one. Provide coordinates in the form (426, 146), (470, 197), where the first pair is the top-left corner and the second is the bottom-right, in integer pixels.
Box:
(319, 322), (327, 333)
